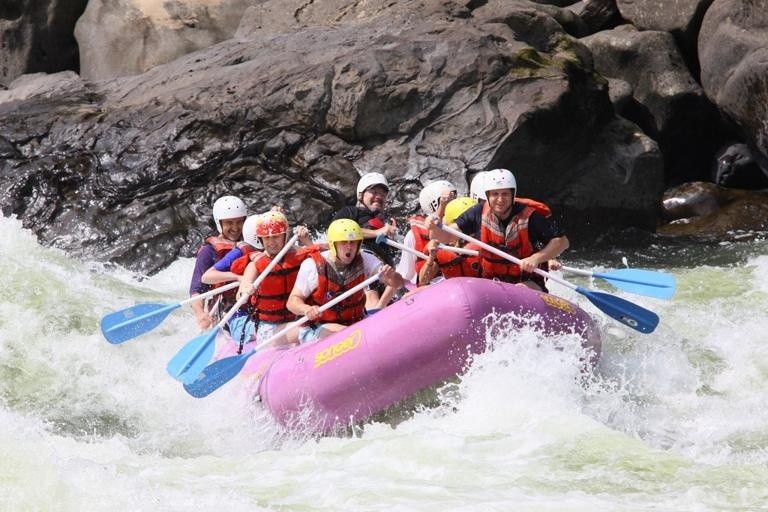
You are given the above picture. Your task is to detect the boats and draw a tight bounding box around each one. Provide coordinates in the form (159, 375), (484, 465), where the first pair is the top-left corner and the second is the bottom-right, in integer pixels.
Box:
(207, 268), (605, 439)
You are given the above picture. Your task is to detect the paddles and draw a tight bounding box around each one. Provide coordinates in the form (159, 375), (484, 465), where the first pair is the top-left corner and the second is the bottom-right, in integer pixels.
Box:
(438, 223), (658, 333)
(438, 237), (676, 300)
(165, 237), (306, 386)
(100, 282), (240, 344)
(183, 201), (377, 397)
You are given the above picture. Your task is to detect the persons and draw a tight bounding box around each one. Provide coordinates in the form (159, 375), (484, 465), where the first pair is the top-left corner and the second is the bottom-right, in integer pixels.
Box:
(330, 169), (570, 310)
(189, 196), (404, 350)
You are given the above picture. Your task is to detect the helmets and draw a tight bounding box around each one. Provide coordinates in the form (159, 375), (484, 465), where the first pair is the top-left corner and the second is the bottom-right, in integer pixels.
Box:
(418, 168), (516, 226)
(242, 211), (288, 251)
(212, 196), (247, 234)
(327, 218), (363, 263)
(356, 172), (388, 201)
